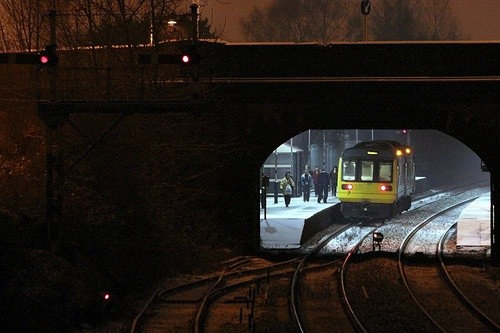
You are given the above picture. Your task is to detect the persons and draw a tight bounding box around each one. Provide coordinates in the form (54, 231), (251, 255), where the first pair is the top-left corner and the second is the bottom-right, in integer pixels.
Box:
(256, 164), (337, 204)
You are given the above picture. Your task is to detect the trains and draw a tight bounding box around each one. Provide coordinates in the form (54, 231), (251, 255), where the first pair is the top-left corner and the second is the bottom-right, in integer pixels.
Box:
(337, 137), (414, 224)
